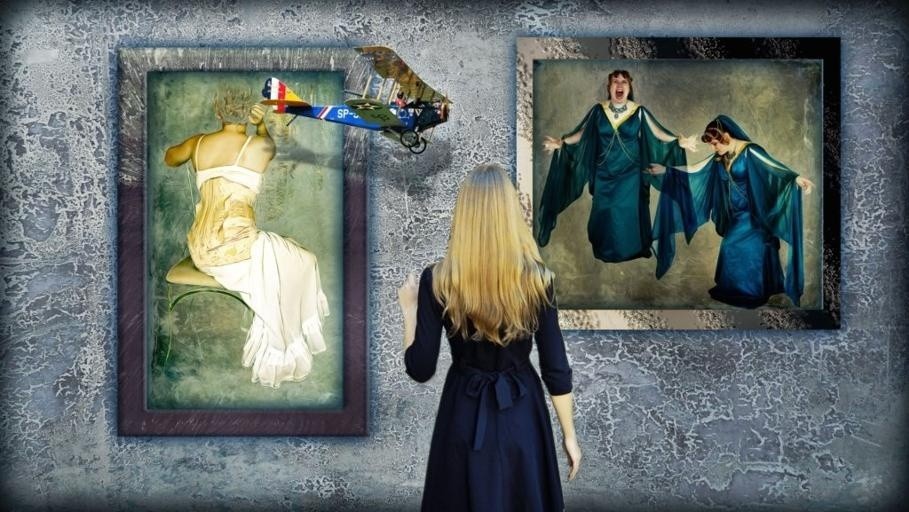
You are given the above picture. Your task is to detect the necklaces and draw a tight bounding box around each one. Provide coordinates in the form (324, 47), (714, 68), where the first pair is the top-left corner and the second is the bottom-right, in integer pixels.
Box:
(608, 102), (627, 118)
(726, 140), (737, 166)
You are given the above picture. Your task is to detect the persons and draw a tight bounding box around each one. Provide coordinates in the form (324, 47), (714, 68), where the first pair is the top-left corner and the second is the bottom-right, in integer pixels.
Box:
(642, 114), (815, 310)
(538, 70), (700, 263)
(397, 163), (581, 512)
(165, 86), (330, 387)
(395, 91), (406, 107)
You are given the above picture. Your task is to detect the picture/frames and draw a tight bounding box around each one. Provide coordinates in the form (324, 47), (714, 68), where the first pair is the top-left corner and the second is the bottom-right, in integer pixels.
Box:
(114, 44), (372, 437)
(514, 33), (844, 332)
